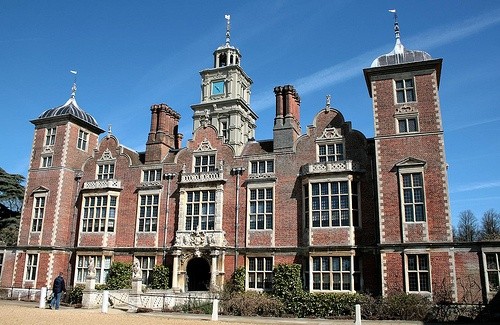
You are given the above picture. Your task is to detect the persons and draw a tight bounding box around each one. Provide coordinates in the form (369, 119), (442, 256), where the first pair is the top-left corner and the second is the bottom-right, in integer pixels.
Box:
(48, 272), (66, 310)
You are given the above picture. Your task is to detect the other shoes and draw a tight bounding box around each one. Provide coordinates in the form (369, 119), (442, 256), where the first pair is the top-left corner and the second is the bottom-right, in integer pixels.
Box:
(49, 304), (52, 309)
(56, 308), (59, 310)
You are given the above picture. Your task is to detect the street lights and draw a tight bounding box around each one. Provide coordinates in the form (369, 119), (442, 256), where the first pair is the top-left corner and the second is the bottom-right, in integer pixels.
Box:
(231, 167), (245, 287)
(163, 173), (175, 267)
